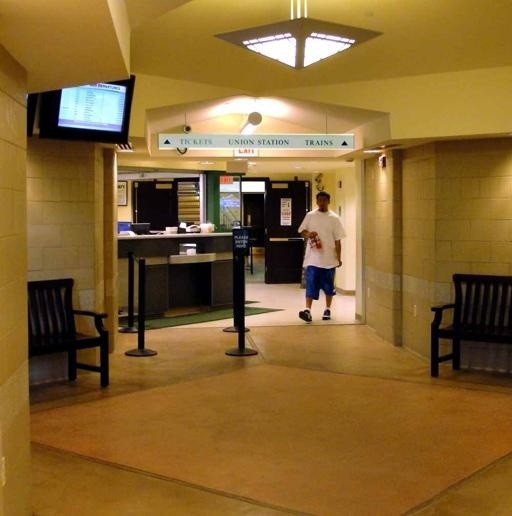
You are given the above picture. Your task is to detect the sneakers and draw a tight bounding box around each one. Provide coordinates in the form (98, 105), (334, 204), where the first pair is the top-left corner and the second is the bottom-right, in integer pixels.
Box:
(322, 309), (331, 321)
(298, 309), (312, 322)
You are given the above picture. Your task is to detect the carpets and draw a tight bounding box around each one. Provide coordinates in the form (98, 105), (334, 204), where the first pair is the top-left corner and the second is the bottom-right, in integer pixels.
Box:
(120, 307), (285, 331)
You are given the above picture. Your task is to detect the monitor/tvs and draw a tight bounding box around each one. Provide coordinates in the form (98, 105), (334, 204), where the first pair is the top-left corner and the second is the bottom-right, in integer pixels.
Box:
(39, 75), (135, 144)
(130, 223), (151, 234)
(118, 222), (132, 234)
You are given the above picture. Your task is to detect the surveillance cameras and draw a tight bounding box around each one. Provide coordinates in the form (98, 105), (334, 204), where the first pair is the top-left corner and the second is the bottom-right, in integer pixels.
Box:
(183, 125), (191, 134)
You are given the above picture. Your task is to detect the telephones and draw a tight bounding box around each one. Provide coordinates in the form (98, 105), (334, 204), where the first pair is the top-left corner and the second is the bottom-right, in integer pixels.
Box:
(186, 225), (201, 233)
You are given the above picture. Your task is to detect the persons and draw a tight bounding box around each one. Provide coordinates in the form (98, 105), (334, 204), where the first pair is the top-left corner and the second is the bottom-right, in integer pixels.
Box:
(297, 191), (348, 322)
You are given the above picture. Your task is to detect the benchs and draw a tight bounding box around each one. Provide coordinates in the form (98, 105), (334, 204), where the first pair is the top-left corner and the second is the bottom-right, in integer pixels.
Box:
(27, 278), (112, 387)
(430, 273), (512, 378)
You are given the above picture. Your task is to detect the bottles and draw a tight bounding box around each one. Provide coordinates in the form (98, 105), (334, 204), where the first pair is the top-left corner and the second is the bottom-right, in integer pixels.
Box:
(315, 235), (321, 248)
